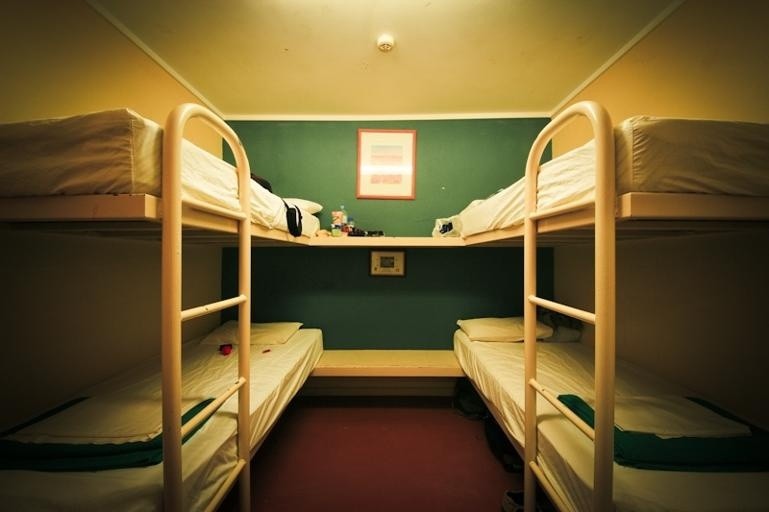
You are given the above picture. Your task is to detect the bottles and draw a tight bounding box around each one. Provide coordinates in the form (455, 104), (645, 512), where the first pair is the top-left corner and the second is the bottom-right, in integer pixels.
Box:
(331, 205), (353, 237)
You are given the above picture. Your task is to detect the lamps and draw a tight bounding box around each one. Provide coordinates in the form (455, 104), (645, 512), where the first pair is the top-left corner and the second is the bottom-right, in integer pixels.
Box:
(377, 32), (396, 53)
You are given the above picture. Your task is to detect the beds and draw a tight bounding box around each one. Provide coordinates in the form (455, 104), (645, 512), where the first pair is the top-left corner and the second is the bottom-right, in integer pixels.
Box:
(0, 102), (326, 511)
(450, 102), (767, 511)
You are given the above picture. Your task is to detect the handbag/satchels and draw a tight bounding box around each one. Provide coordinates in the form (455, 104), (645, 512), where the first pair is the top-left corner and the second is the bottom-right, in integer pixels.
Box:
(252, 172), (275, 193)
